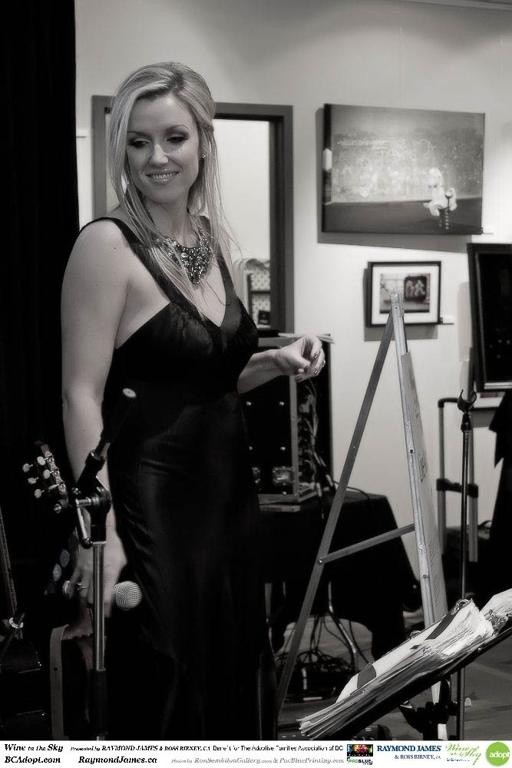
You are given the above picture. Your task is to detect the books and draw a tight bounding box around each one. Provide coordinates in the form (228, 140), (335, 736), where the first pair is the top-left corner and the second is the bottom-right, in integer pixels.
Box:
(296, 588), (512, 740)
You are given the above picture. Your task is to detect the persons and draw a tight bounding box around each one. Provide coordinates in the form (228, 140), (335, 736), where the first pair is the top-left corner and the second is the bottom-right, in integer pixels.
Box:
(60, 59), (328, 739)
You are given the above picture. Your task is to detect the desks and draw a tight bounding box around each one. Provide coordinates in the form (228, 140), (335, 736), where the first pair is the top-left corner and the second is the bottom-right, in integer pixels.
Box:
(256, 490), (425, 703)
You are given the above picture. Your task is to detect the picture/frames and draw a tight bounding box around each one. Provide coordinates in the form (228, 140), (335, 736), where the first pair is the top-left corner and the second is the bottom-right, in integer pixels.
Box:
(467, 243), (512, 393)
(364, 261), (444, 328)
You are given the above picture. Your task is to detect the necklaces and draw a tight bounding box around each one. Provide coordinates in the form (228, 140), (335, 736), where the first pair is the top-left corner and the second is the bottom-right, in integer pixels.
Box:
(152, 217), (215, 287)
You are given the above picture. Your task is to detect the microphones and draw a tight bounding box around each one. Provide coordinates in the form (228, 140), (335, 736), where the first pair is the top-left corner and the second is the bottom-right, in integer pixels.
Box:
(62, 580), (142, 610)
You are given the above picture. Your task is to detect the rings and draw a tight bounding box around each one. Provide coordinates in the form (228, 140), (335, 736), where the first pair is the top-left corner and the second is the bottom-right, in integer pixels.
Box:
(76, 582), (87, 592)
